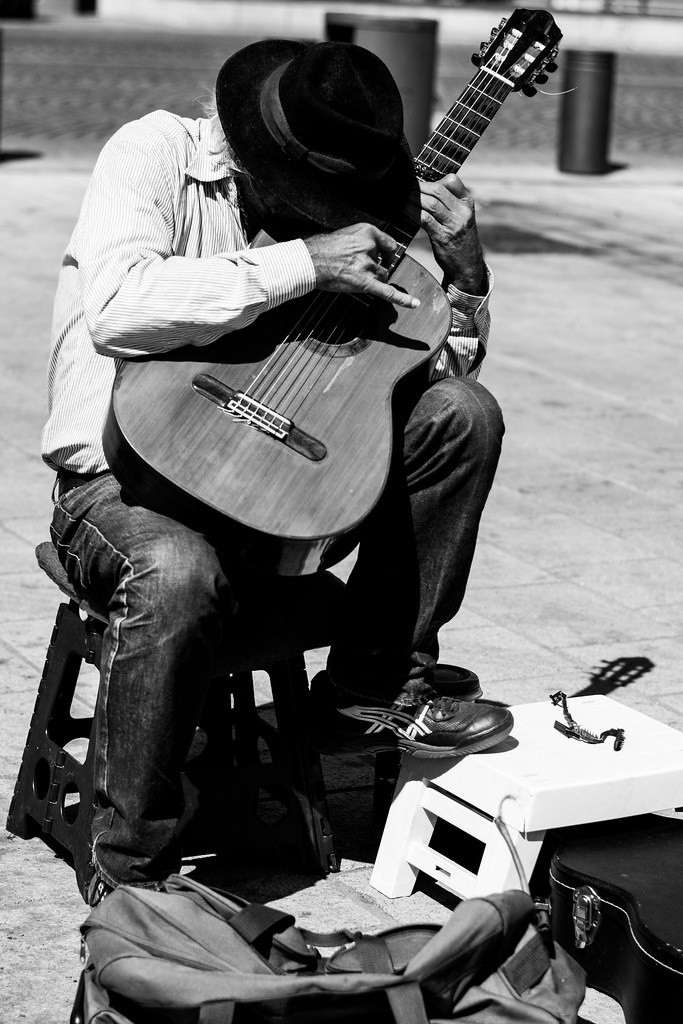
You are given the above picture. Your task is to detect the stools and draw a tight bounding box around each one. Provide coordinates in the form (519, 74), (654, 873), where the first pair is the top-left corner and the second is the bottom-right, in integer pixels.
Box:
(368, 694), (682, 901)
(6, 540), (349, 905)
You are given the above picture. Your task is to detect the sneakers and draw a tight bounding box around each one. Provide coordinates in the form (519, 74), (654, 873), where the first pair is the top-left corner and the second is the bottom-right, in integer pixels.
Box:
(86, 866), (166, 910)
(306, 669), (515, 760)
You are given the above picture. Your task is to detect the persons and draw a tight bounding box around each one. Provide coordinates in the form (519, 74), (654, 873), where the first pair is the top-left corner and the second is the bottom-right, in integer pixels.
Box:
(42, 35), (515, 905)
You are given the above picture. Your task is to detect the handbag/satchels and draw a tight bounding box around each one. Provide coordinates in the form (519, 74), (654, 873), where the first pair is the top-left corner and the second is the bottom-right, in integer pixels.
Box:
(66, 873), (588, 1023)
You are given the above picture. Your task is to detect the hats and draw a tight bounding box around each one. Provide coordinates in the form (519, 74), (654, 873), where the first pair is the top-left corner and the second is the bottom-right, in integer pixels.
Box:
(216, 37), (419, 240)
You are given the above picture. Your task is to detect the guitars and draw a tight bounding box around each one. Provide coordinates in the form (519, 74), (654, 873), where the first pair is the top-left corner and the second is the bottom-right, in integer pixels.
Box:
(101, 7), (569, 580)
